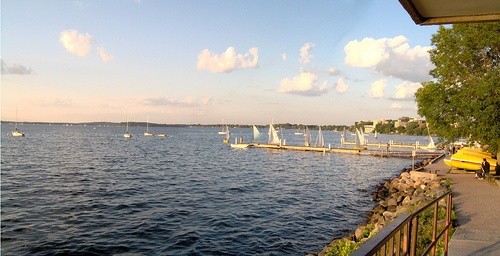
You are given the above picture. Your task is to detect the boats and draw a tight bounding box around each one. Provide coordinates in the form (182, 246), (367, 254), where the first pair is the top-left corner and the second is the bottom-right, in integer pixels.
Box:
(11, 128), (24, 137)
(158, 133), (167, 136)
(444, 147), (498, 172)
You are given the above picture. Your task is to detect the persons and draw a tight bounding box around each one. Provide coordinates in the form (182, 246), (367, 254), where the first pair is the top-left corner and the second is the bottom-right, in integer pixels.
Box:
(475, 158), (490, 180)
(444, 145), (455, 155)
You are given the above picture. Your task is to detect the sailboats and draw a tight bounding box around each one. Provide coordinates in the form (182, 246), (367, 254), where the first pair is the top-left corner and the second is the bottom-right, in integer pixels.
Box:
(143, 118), (154, 136)
(355, 126), (368, 150)
(341, 126), (357, 145)
(230, 135), (249, 149)
(374, 130), (378, 139)
(123, 120), (133, 138)
(303, 124), (326, 152)
(266, 124), (280, 148)
(218, 124), (230, 143)
